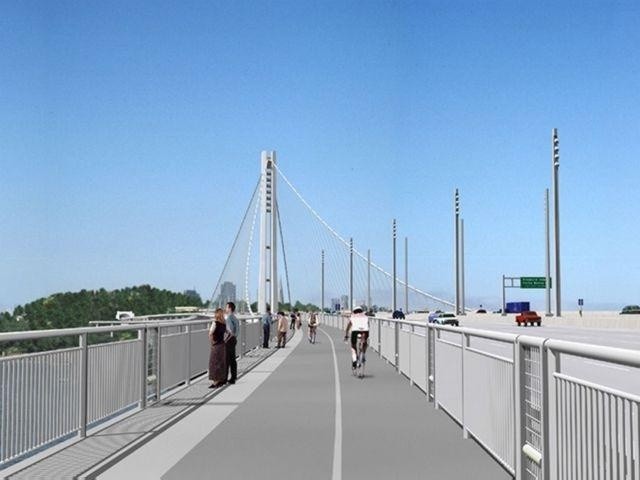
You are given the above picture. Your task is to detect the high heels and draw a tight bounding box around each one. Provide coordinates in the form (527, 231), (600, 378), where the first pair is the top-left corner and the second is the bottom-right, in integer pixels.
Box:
(208, 381), (223, 388)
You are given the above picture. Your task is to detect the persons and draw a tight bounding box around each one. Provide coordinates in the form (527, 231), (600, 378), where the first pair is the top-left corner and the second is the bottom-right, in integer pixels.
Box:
(290, 311), (295, 330)
(296, 312), (301, 329)
(261, 307), (271, 349)
(344, 306), (370, 368)
(209, 308), (233, 388)
(275, 312), (288, 348)
(224, 302), (240, 382)
(308, 313), (318, 341)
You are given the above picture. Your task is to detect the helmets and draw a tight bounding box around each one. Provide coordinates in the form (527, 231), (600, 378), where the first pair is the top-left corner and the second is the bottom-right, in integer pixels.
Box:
(353, 306), (363, 314)
